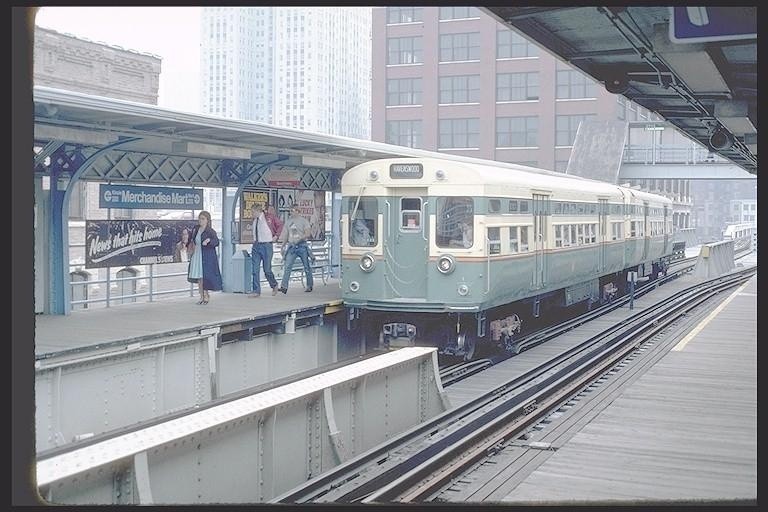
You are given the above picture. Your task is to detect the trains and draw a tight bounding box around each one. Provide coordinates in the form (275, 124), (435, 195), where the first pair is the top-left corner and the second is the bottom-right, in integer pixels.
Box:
(340, 156), (673, 363)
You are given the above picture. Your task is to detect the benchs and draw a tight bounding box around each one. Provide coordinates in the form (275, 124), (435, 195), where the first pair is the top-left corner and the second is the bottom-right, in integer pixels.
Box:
(279, 249), (342, 290)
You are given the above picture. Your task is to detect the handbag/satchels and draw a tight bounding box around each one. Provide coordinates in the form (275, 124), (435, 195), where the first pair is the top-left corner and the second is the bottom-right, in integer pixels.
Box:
(188, 242), (195, 255)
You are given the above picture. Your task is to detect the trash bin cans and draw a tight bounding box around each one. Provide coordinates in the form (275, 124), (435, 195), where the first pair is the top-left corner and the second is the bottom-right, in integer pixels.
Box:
(231, 250), (252, 294)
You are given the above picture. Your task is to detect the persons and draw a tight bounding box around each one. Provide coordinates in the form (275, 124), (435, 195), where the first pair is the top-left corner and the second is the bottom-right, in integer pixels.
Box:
(447, 218), (473, 248)
(246, 203), (283, 298)
(187, 211), (222, 305)
(172, 229), (190, 262)
(278, 203), (314, 294)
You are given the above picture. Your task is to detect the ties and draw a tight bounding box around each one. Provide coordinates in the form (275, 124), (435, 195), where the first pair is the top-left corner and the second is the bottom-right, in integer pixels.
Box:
(255, 218), (259, 242)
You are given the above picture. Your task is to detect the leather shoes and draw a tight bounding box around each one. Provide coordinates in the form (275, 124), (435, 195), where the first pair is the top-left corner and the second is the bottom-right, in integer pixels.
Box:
(197, 295), (210, 305)
(247, 285), (312, 297)
